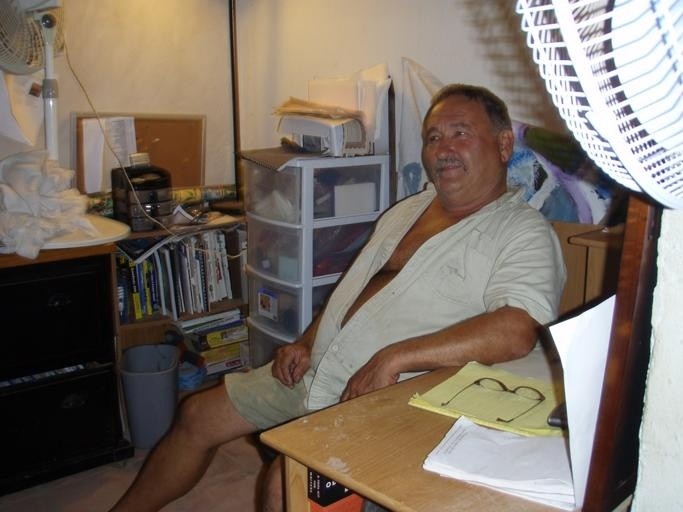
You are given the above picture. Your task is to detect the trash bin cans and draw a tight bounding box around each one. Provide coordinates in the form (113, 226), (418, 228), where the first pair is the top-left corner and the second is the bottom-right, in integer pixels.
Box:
(116, 344), (180, 450)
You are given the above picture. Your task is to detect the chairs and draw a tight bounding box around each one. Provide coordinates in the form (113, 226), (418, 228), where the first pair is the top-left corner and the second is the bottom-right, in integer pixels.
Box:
(550, 213), (624, 337)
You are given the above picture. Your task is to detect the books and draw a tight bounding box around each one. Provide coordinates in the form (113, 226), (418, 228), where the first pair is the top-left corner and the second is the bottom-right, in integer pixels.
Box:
(238, 139), (327, 175)
(408, 359), (570, 440)
(422, 415), (577, 510)
(176, 309), (250, 380)
(116, 231), (233, 323)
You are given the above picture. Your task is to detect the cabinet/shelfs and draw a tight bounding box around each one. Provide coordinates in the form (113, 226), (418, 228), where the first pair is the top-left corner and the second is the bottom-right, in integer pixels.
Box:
(236, 154), (389, 374)
(0, 237), (127, 494)
(102, 209), (258, 431)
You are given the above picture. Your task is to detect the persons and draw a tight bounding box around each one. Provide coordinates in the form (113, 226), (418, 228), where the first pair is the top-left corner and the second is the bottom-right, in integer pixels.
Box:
(111, 83), (569, 512)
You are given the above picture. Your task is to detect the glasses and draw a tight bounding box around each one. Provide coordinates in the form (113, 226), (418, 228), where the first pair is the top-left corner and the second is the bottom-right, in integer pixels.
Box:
(442, 377), (546, 422)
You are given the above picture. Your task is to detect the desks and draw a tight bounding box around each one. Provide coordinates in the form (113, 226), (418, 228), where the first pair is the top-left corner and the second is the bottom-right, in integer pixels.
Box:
(259, 360), (627, 511)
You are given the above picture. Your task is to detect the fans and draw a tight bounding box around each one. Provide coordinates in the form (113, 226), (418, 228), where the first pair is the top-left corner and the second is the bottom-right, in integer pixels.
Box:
(514, 0), (683, 209)
(0, 0), (131, 248)
